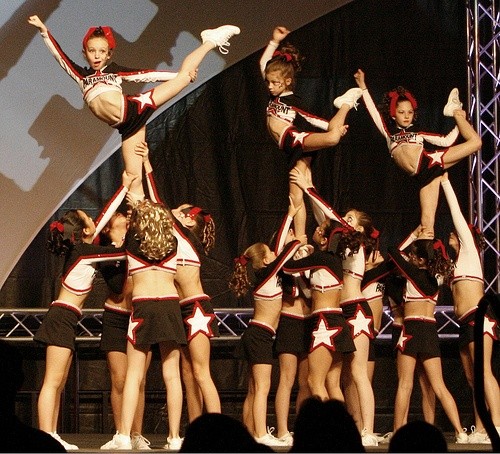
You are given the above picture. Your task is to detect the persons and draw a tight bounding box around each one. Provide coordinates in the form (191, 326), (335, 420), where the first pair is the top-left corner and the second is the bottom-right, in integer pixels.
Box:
(34, 139), (500, 449)
(0, 423), (69, 454)
(179, 412), (260, 454)
(351, 63), (482, 251)
(259, 24), (363, 245)
(27, 10), (242, 218)
(386, 420), (449, 454)
(286, 394), (367, 454)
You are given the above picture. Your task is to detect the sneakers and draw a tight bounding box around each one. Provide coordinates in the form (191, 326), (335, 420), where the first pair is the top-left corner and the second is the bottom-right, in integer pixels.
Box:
(166, 436), (181, 450)
(132, 432), (152, 451)
(101, 433), (131, 450)
(442, 88), (464, 116)
(333, 88), (363, 111)
(276, 431), (294, 446)
(254, 426), (285, 446)
(200, 25), (239, 55)
(361, 428), (378, 446)
(457, 429), (467, 444)
(50, 431), (79, 451)
(468, 426), (491, 444)
